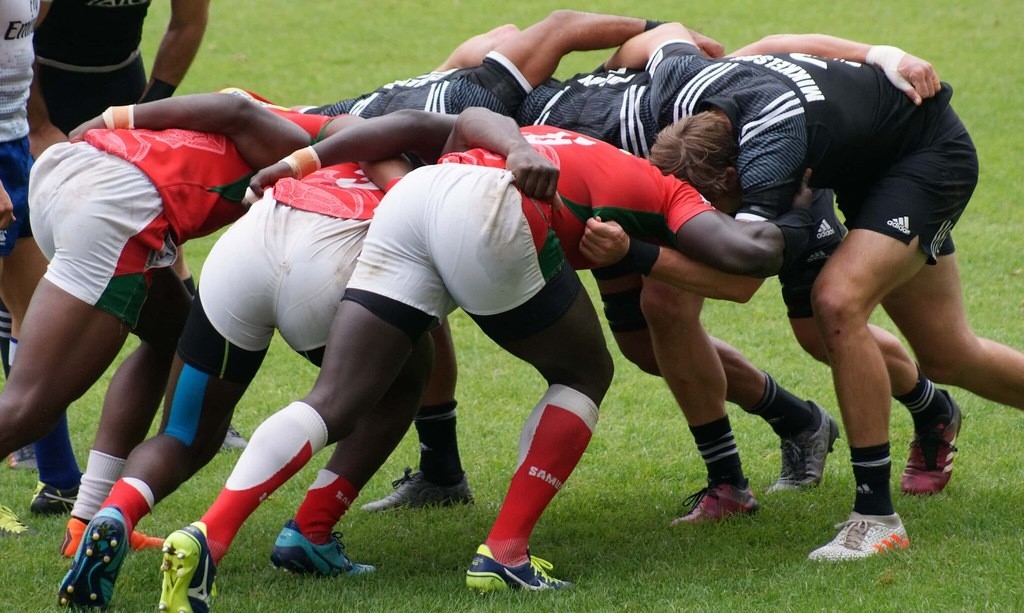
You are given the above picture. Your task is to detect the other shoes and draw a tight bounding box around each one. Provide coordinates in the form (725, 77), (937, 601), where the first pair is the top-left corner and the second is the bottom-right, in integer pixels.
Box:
(8, 447), (39, 472)
(29, 473), (85, 515)
(0, 503), (37, 538)
(61, 515), (166, 558)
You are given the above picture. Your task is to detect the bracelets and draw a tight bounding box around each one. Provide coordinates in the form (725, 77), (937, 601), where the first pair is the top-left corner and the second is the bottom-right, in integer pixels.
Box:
(101, 105), (135, 131)
(139, 78), (175, 104)
(280, 146), (322, 182)
(610, 237), (660, 281)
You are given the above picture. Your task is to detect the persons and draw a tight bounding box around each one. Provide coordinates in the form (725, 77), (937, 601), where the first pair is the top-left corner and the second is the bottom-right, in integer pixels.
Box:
(0, 10), (1024, 613)
(0, 0), (213, 467)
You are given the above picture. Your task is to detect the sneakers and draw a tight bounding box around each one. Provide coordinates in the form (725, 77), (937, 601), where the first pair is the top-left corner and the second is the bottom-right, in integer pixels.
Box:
(809, 512), (909, 562)
(901, 389), (962, 493)
(57, 506), (128, 613)
(361, 467), (474, 513)
(466, 544), (573, 595)
(159, 522), (218, 613)
(670, 478), (760, 529)
(271, 521), (376, 580)
(768, 401), (839, 490)
